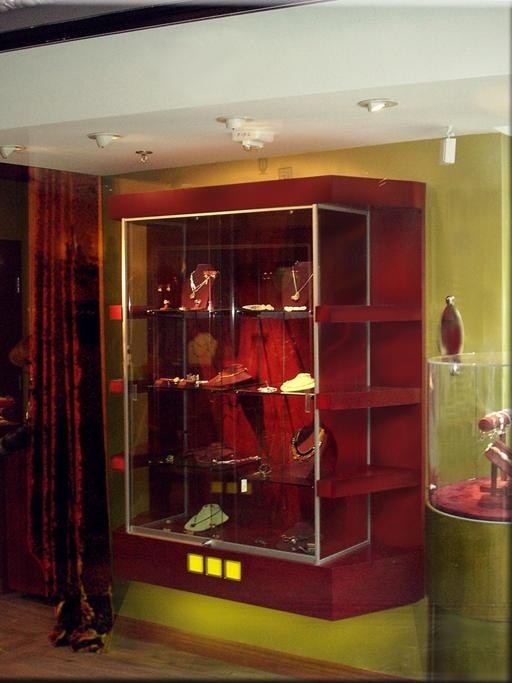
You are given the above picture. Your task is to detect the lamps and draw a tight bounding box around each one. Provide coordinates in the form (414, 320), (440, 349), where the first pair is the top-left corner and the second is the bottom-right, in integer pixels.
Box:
(439, 128), (457, 165)
(359, 98), (399, 113)
(439, 295), (463, 356)
(87, 131), (122, 149)
(0, 145), (25, 159)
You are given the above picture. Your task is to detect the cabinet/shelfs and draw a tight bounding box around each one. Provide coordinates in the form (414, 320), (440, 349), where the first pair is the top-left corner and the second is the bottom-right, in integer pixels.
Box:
(423, 352), (512, 519)
(101, 171), (426, 623)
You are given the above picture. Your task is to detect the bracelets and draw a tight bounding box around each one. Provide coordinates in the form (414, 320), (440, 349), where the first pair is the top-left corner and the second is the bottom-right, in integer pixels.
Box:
(479, 408), (512, 441)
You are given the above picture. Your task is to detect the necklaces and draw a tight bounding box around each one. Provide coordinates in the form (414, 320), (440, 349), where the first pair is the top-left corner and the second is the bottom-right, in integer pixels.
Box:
(215, 367), (249, 383)
(289, 426), (325, 463)
(190, 508), (222, 527)
(291, 266), (313, 301)
(192, 338), (213, 358)
(189, 271), (210, 299)
(283, 379), (315, 388)
